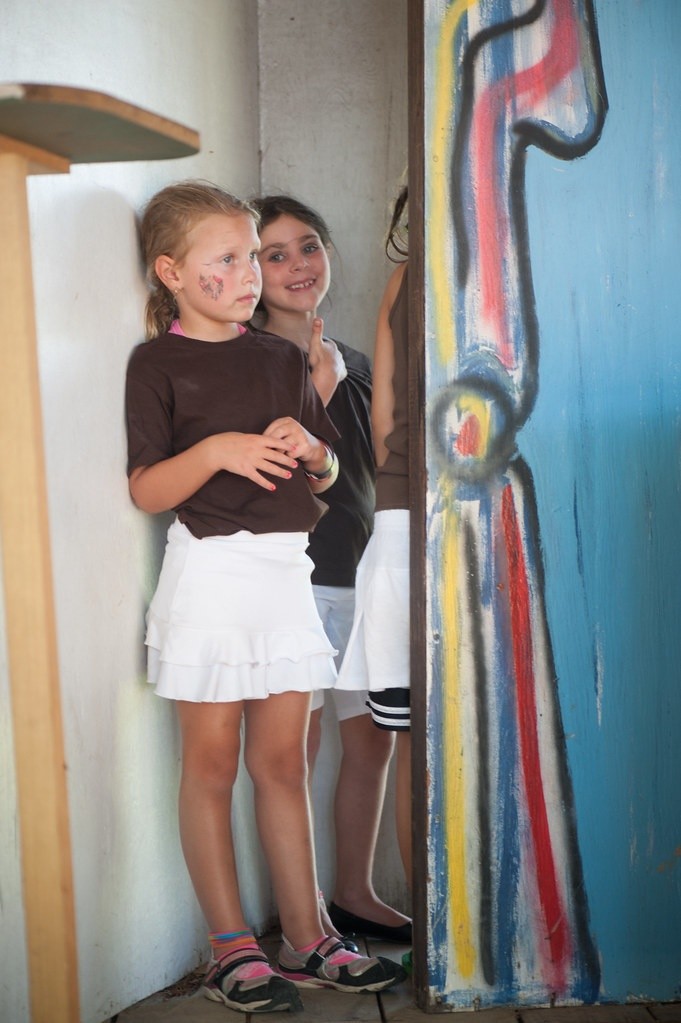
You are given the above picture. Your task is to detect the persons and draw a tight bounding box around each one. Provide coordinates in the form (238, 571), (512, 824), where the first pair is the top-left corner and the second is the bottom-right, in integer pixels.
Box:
(333, 164), (411, 897)
(125, 180), (409, 1013)
(246, 194), (414, 954)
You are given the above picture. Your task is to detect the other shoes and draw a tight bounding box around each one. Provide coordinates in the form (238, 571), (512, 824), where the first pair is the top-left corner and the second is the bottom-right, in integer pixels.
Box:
(340, 936), (360, 953)
(329, 901), (413, 945)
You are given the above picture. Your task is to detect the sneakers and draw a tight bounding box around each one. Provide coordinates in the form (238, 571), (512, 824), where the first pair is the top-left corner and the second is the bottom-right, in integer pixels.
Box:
(274, 935), (406, 995)
(201, 947), (306, 1014)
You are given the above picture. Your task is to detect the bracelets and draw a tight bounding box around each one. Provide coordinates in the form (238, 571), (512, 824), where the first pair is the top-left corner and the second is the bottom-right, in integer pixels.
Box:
(303, 432), (336, 482)
